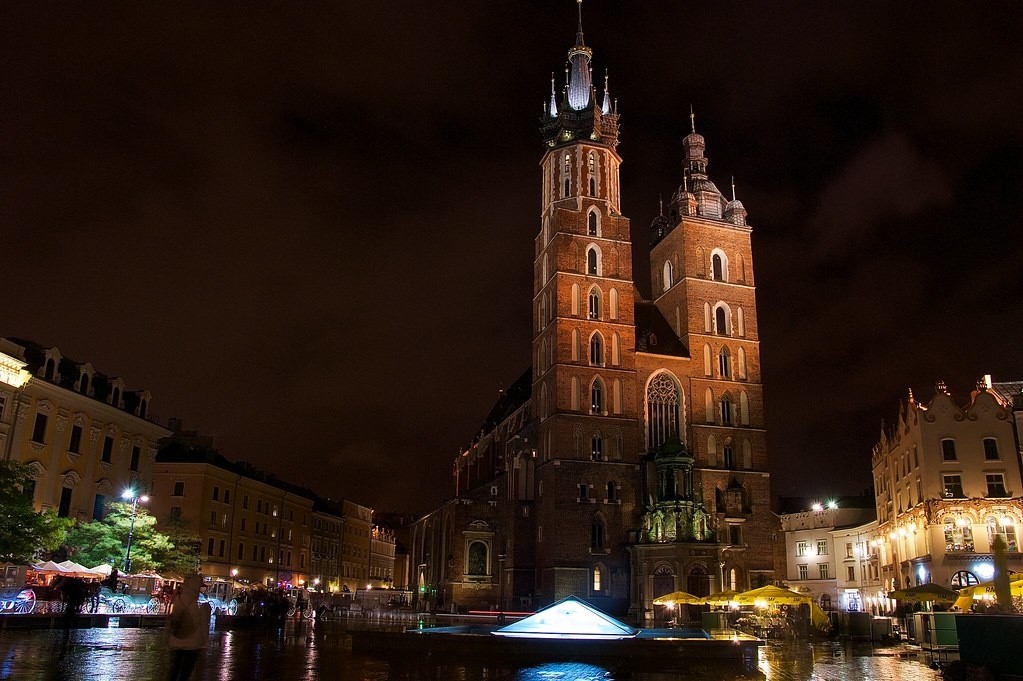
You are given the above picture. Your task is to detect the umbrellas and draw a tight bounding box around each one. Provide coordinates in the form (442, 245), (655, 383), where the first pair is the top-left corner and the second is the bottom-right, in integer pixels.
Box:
(887, 583), (959, 613)
(653, 585), (811, 618)
(36, 560), (333, 594)
(959, 572), (1023, 597)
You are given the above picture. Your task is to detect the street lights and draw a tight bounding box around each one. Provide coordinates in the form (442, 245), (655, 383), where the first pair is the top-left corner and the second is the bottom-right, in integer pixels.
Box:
(121, 488), (152, 593)
(315, 578), (319, 592)
(230, 569), (239, 597)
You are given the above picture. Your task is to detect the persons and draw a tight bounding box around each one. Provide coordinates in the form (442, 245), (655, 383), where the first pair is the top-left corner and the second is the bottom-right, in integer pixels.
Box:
(298, 590), (303, 598)
(122, 580), (126, 595)
(317, 605), (331, 631)
(163, 573), (209, 681)
(100, 567), (119, 591)
(31, 578), (38, 584)
(341, 585), (351, 599)
(163, 581), (183, 614)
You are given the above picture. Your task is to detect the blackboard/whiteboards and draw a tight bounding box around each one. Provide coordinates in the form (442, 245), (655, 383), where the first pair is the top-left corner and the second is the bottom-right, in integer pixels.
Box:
(849, 612), (872, 638)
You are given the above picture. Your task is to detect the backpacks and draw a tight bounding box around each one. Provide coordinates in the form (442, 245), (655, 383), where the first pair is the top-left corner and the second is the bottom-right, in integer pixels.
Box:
(174, 595), (196, 639)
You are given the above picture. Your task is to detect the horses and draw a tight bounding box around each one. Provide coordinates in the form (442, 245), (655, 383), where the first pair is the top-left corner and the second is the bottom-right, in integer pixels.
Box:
(49, 574), (100, 615)
